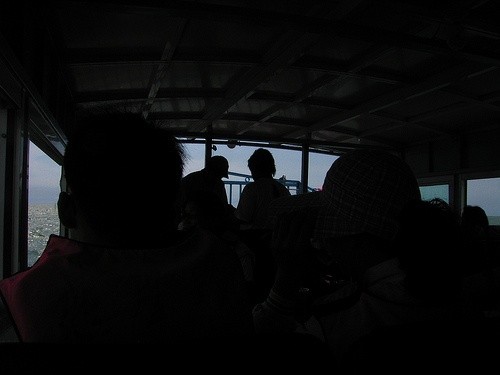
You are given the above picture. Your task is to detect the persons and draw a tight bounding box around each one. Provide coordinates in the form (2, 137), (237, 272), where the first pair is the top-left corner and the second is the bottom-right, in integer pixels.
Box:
(262, 144), (440, 359)
(184, 190), (258, 292)
(236, 148), (291, 223)
(0, 112), (244, 344)
(180, 153), (236, 215)
(460, 207), (500, 268)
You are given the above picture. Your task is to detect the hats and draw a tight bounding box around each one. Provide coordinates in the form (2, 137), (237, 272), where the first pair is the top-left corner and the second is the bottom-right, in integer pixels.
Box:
(265, 155), (409, 239)
(208, 156), (228, 178)
